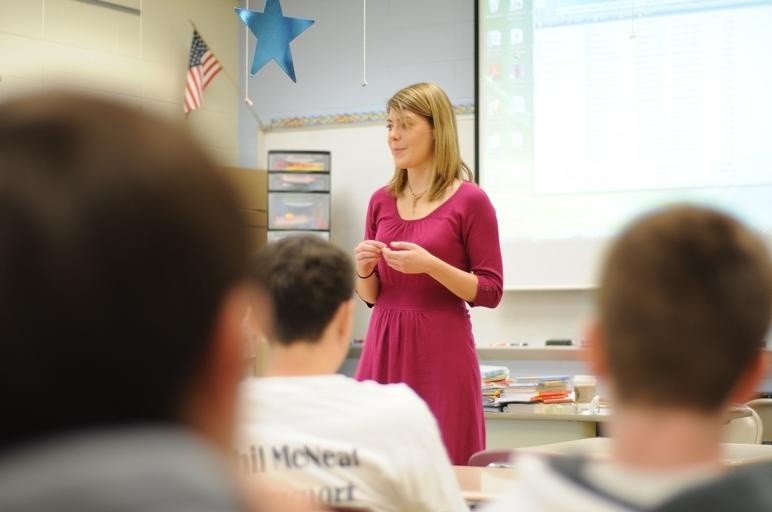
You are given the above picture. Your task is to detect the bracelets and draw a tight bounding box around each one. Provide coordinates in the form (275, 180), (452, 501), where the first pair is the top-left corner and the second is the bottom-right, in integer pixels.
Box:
(356, 268), (375, 279)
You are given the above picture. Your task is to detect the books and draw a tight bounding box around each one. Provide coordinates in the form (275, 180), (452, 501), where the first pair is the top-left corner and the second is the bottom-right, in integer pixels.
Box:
(478, 364), (610, 414)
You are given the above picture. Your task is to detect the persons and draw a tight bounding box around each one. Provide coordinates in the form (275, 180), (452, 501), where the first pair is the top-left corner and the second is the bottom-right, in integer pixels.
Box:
(352, 80), (505, 467)
(235, 229), (477, 512)
(0, 88), (331, 512)
(481, 198), (772, 511)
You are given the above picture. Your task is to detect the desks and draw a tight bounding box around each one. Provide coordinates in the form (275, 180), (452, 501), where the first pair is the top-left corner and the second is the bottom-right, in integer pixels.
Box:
(451, 465), (520, 511)
(483, 397), (613, 450)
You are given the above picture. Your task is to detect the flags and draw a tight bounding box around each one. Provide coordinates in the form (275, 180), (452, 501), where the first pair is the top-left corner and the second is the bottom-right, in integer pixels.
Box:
(183, 28), (223, 120)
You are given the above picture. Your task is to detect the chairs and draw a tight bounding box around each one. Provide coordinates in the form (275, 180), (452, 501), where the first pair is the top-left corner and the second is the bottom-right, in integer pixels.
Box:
(746, 396), (772, 446)
(723, 402), (763, 445)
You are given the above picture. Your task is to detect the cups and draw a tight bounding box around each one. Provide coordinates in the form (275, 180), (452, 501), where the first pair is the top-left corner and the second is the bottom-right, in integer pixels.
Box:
(572, 373), (601, 410)
